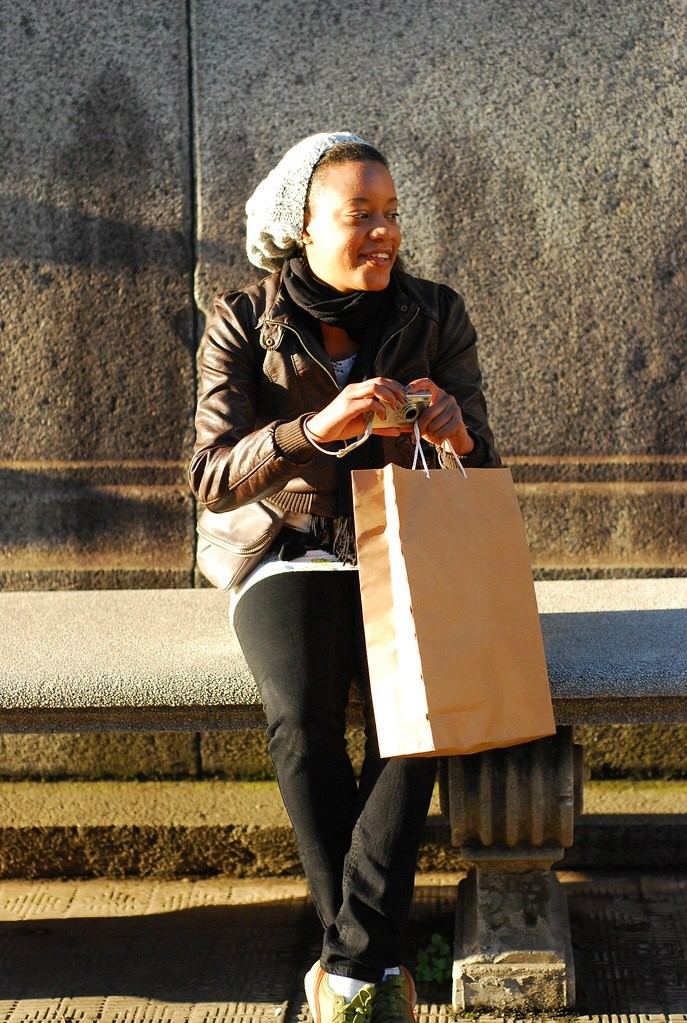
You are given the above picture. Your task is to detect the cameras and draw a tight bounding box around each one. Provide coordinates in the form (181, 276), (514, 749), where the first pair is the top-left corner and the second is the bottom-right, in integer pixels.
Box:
(363, 389), (433, 428)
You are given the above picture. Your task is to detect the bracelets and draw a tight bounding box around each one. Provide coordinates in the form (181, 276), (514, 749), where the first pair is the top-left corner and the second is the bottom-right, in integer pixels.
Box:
(435, 444), (468, 467)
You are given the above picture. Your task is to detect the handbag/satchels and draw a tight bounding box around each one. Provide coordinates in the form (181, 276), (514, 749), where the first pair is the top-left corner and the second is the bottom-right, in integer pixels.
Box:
(196, 499), (288, 593)
(351, 420), (557, 760)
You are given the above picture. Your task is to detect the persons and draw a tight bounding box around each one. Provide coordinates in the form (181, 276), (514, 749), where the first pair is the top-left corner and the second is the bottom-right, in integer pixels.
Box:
(189, 130), (503, 1023)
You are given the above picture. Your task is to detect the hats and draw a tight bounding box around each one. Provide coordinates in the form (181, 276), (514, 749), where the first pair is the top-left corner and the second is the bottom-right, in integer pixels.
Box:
(244, 132), (374, 274)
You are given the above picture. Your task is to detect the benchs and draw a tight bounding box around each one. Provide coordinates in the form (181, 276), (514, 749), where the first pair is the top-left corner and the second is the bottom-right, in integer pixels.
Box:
(1, 580), (687, 1011)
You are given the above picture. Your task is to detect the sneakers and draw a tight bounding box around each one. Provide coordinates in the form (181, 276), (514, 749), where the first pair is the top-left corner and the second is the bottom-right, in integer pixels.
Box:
(304, 958), (378, 1023)
(370, 963), (417, 1023)
